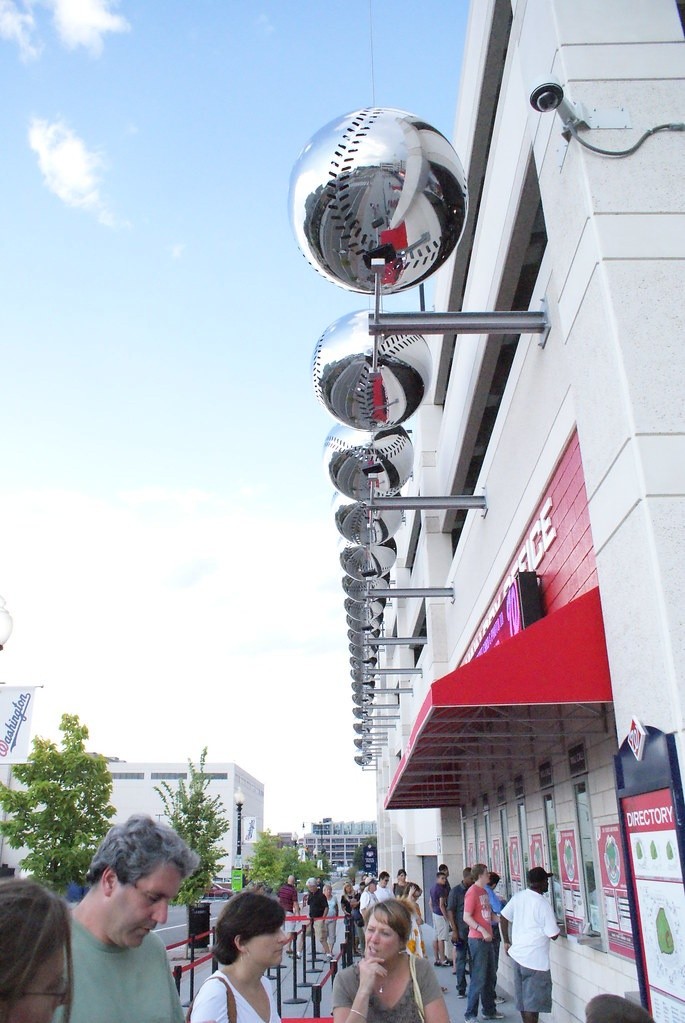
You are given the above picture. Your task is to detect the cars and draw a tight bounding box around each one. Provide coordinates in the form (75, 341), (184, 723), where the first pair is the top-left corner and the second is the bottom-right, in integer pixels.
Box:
(202, 883), (233, 899)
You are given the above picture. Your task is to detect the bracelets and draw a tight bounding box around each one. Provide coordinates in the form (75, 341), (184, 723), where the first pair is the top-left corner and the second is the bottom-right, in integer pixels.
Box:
(475, 923), (480, 931)
(351, 1009), (367, 1020)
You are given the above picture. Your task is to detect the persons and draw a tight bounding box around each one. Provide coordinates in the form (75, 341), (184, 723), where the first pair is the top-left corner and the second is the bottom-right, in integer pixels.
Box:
(0, 877), (77, 1023)
(278, 864), (656, 1023)
(189, 891), (287, 1023)
(48, 815), (202, 1023)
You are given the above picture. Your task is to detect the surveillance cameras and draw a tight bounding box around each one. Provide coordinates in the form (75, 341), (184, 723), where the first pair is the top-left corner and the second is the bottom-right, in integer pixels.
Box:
(527, 75), (564, 113)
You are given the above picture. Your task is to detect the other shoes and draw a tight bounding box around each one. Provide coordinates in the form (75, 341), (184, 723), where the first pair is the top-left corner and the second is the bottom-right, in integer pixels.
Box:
(326, 955), (333, 961)
(494, 996), (506, 1004)
(286, 950), (293, 953)
(465, 1017), (480, 1023)
(482, 1012), (505, 1020)
(457, 994), (467, 999)
(289, 955), (302, 959)
(452, 968), (470, 975)
(440, 959), (454, 967)
(433, 960), (441, 966)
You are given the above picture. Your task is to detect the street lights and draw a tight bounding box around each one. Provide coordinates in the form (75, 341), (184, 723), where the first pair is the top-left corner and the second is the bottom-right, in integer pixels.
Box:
(302, 822), (325, 871)
(291, 832), (298, 887)
(233, 787), (246, 870)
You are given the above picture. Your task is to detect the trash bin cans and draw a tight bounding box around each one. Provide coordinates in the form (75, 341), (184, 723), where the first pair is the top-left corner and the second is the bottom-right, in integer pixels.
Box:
(188, 902), (211, 948)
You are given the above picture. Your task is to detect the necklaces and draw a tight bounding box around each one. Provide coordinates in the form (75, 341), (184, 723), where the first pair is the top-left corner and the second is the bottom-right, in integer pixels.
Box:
(379, 958), (402, 994)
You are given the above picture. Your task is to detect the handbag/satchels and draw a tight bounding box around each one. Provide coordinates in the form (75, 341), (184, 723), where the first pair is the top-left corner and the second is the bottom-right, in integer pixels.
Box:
(350, 909), (364, 927)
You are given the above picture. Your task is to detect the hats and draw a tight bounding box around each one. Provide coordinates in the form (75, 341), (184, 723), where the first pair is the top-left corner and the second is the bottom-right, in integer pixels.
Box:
(584, 994), (655, 1023)
(365, 877), (379, 886)
(528, 867), (553, 882)
